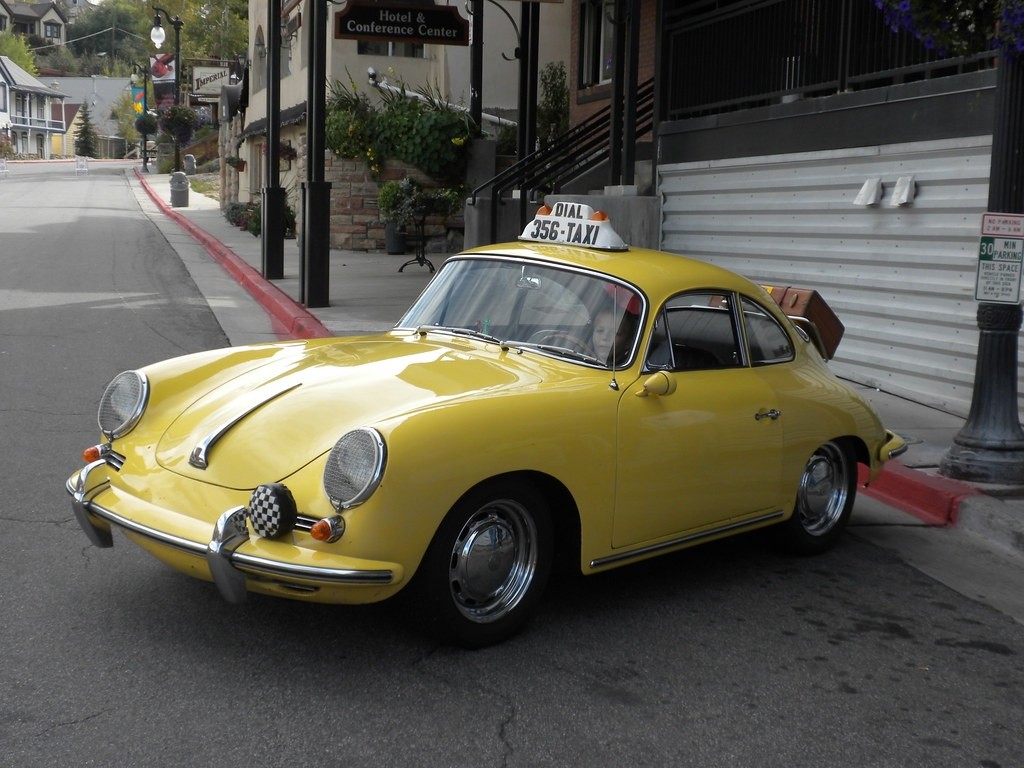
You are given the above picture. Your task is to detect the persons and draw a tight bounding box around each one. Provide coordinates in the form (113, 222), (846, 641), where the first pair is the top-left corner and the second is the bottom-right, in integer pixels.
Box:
(592, 306), (634, 366)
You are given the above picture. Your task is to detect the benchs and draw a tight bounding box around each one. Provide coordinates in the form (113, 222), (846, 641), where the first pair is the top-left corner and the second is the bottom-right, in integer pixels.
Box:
(648, 341), (718, 372)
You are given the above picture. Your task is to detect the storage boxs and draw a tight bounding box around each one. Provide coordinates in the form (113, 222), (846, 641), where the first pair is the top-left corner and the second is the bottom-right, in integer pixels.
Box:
(707, 284), (845, 360)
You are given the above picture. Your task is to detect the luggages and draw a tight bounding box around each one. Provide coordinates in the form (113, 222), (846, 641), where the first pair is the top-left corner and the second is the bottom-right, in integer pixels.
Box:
(709, 284), (845, 360)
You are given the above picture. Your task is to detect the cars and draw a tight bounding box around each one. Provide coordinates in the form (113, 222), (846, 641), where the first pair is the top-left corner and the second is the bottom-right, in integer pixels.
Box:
(60, 200), (911, 653)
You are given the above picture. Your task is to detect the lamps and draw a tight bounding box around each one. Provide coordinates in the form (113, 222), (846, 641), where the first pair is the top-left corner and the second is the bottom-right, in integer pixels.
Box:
(280, 18), (298, 45)
(16, 95), (21, 100)
(236, 54), (252, 69)
(254, 37), (267, 63)
(24, 96), (27, 101)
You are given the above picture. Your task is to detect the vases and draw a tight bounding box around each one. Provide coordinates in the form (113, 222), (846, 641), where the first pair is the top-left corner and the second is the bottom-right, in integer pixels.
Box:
(414, 198), (435, 216)
(385, 220), (408, 255)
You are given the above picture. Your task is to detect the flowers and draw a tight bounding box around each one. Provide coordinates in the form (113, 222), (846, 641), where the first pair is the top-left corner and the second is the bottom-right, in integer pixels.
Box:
(135, 114), (158, 137)
(377, 175), (464, 227)
(875, 0), (1024, 54)
(158, 106), (205, 143)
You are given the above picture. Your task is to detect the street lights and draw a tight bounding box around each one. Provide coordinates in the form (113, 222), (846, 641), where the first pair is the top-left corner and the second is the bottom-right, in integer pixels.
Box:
(128, 62), (150, 173)
(148, 4), (185, 173)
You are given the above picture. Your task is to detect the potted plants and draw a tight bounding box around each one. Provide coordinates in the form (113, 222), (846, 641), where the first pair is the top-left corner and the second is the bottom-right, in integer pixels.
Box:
(245, 169), (298, 238)
(261, 141), (297, 161)
(225, 155), (247, 172)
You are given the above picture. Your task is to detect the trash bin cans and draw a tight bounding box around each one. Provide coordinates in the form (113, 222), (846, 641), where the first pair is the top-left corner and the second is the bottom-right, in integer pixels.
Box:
(184, 153), (197, 175)
(170, 172), (189, 207)
